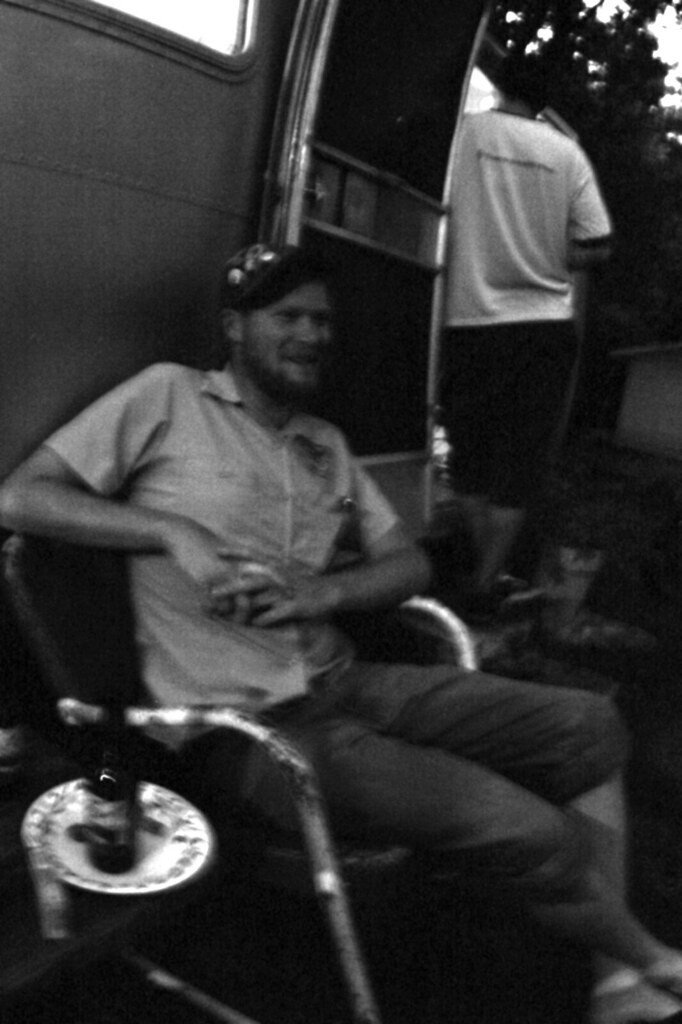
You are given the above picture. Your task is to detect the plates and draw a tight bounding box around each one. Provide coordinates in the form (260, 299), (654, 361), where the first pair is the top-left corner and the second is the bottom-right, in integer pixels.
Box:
(19, 776), (215, 895)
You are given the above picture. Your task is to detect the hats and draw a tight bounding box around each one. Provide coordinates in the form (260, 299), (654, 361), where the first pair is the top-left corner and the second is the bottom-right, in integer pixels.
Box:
(223, 234), (331, 315)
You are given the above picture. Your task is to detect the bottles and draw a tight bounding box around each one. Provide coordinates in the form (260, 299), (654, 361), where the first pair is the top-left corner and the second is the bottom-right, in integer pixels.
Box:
(85, 688), (138, 873)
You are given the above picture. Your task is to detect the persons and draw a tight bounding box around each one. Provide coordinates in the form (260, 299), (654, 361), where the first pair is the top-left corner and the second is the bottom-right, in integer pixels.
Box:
(0, 238), (682, 1024)
(443, 54), (614, 619)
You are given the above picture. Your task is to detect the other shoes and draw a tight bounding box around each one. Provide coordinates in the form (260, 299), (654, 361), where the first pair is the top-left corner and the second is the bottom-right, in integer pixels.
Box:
(584, 951), (682, 1023)
(466, 578), (508, 611)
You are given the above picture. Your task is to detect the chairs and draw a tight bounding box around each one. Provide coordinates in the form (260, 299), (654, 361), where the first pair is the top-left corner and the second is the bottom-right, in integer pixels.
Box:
(0, 533), (480, 1024)
